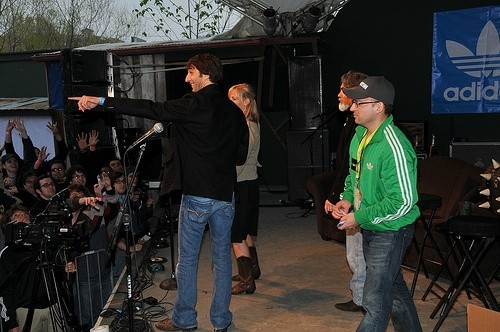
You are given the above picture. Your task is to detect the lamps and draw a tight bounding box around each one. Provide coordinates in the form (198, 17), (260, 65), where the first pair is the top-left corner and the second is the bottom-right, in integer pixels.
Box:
(263, 8), (277, 35)
(302, 7), (322, 32)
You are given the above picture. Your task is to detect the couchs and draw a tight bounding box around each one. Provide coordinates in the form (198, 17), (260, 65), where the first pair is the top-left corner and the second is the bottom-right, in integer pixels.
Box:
(306, 155), (500, 287)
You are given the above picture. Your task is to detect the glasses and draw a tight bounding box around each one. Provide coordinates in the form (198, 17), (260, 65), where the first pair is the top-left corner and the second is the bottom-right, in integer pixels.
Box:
(50, 168), (63, 172)
(352, 100), (380, 107)
(41, 182), (55, 187)
(102, 171), (110, 175)
(71, 175), (86, 179)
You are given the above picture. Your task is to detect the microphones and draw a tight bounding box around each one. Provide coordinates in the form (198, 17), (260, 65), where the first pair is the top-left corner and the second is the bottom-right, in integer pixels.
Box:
(129, 122), (164, 151)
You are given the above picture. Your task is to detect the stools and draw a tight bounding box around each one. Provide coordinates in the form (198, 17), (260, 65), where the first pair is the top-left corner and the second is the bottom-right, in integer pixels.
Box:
(409, 194), (500, 332)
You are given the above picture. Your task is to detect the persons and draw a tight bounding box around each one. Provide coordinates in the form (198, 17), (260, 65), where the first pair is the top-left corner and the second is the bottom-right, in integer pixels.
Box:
(1, 112), (153, 332)
(331, 76), (425, 332)
(325, 70), (372, 312)
(228, 84), (262, 295)
(67, 52), (250, 332)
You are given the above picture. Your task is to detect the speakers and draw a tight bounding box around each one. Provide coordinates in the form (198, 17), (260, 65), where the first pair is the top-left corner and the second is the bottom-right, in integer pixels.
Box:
(61, 48), (117, 150)
(284, 55), (331, 201)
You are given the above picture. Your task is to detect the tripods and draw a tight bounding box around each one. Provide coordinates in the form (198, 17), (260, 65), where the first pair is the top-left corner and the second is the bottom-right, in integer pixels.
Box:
(22, 243), (81, 332)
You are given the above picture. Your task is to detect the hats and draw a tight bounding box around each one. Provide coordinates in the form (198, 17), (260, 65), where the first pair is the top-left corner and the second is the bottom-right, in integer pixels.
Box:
(343, 76), (395, 104)
(1, 154), (20, 162)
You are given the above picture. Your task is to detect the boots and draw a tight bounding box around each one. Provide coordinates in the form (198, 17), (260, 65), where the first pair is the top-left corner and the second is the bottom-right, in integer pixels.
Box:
(232, 246), (261, 281)
(231, 256), (256, 294)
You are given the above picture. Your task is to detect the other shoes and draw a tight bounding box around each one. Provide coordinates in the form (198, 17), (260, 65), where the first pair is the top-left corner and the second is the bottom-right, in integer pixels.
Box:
(114, 179), (124, 184)
(155, 318), (197, 332)
(334, 300), (367, 313)
(214, 310), (233, 332)
